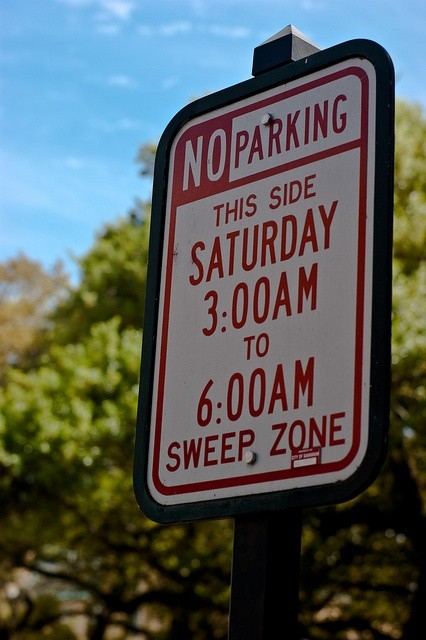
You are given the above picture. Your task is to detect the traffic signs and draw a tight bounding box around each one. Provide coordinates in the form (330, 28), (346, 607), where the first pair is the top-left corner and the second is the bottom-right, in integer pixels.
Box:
(134, 39), (395, 521)
